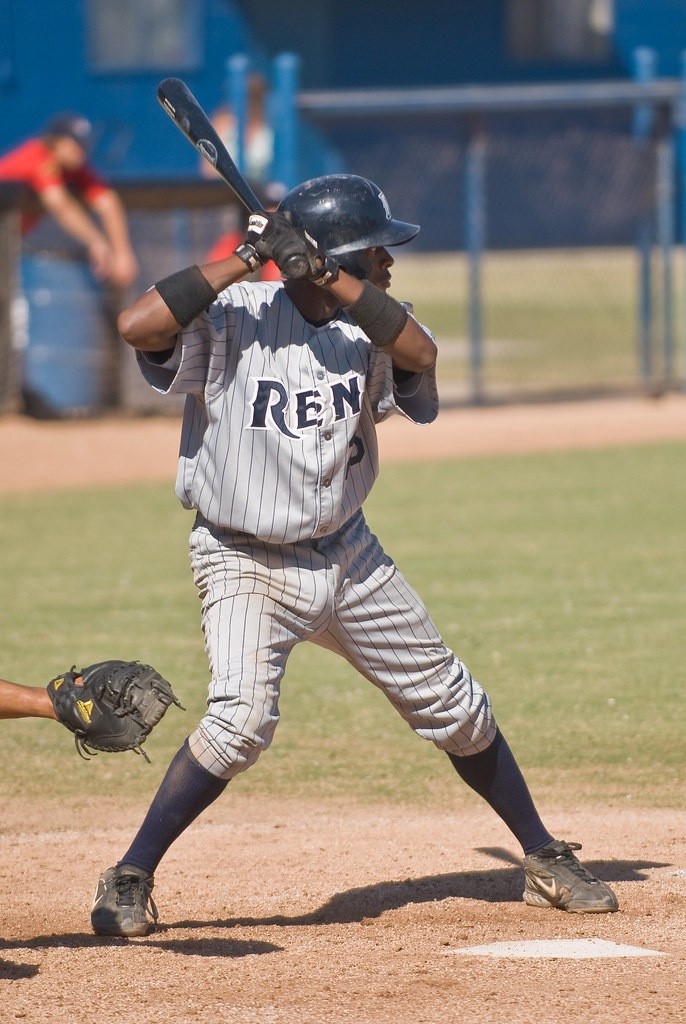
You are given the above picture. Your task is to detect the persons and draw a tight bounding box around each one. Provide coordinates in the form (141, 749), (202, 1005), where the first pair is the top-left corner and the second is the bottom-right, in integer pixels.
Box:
(93, 175), (616, 938)
(0, 65), (291, 297)
(0, 660), (188, 763)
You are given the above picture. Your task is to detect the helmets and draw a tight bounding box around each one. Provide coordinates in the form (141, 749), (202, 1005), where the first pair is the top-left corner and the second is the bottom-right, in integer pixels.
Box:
(273, 174), (420, 256)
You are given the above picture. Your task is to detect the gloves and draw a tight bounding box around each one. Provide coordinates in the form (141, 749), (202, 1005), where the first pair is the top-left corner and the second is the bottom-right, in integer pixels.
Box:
(246, 211), (338, 288)
(235, 243), (269, 272)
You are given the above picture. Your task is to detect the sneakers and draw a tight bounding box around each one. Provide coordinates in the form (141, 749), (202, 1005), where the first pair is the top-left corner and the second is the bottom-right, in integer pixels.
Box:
(91, 864), (159, 936)
(522, 840), (619, 913)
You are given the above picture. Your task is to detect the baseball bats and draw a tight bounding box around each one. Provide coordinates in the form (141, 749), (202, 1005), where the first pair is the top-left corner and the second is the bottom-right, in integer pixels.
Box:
(149, 67), (313, 284)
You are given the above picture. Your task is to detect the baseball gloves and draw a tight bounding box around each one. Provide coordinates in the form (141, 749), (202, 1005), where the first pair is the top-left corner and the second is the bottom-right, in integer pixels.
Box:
(40, 650), (183, 764)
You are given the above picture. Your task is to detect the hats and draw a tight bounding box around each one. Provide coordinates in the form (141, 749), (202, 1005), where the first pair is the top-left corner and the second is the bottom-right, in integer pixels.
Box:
(51, 117), (91, 152)
